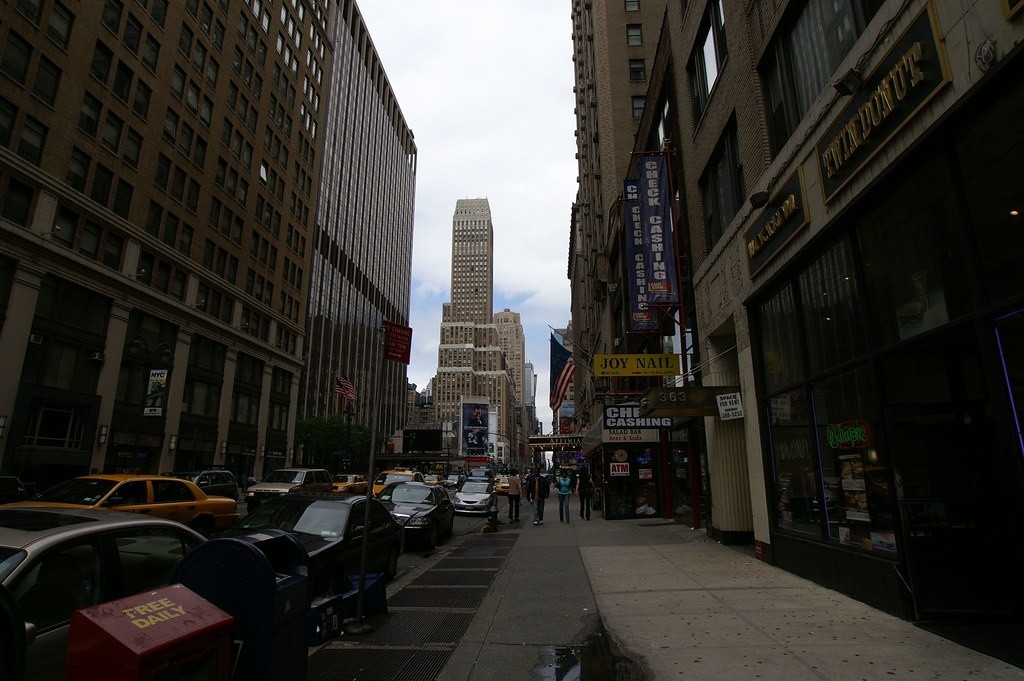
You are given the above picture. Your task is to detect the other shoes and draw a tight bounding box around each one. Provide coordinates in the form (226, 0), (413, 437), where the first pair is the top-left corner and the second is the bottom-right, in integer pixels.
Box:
(508, 499), (592, 526)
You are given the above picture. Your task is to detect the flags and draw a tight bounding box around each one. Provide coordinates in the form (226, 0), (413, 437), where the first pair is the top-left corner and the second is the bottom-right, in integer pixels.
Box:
(335, 377), (356, 400)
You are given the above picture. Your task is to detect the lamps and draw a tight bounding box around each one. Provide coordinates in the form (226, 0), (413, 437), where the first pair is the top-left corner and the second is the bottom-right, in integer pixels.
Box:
(749, 190), (771, 209)
(832, 63), (868, 95)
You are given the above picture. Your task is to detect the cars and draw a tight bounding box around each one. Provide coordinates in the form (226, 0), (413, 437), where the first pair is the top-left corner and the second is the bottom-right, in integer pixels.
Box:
(0, 466), (533, 681)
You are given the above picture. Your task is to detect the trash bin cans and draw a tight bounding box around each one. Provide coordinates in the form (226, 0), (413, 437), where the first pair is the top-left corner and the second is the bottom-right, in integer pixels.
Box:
(167, 529), (317, 681)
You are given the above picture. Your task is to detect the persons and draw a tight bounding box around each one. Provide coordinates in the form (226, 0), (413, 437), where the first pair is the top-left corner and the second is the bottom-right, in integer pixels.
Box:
(508, 466), (592, 527)
